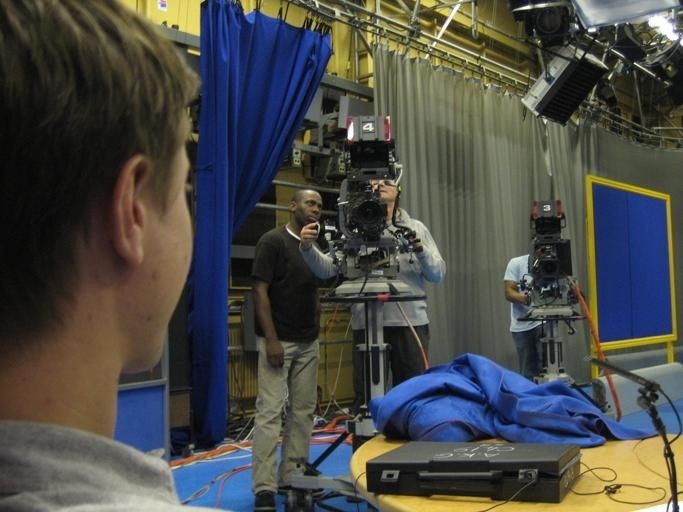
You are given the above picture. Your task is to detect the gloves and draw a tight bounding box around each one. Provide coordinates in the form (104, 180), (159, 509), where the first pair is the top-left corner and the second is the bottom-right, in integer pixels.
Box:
(401, 226), (423, 252)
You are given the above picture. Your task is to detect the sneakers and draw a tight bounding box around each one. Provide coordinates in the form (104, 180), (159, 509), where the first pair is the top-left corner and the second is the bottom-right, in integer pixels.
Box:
(278, 485), (326, 496)
(255, 490), (276, 512)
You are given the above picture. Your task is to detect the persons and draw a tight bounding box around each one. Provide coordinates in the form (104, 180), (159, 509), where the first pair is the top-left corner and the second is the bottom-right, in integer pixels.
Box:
(298, 180), (448, 503)
(502, 253), (546, 383)
(0, 0), (232, 511)
(248, 188), (327, 511)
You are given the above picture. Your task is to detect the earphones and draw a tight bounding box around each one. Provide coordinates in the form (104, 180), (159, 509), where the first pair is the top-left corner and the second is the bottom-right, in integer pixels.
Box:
(398, 185), (402, 193)
